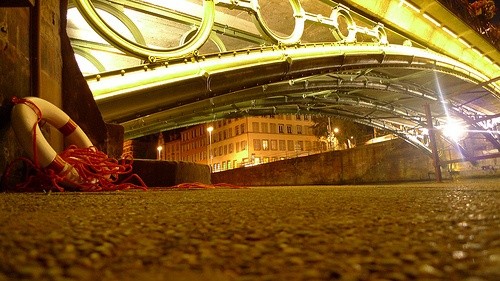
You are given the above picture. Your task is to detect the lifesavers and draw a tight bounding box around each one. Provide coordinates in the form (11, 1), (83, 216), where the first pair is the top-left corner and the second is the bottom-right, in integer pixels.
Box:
(12, 97), (110, 189)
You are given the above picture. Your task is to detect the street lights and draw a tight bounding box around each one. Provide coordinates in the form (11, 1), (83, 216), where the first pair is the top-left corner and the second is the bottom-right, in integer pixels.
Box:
(157, 145), (163, 160)
(207, 126), (214, 166)
